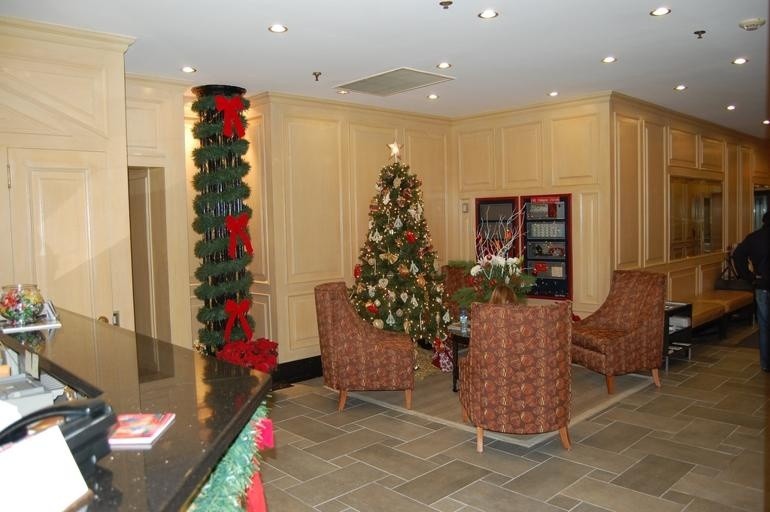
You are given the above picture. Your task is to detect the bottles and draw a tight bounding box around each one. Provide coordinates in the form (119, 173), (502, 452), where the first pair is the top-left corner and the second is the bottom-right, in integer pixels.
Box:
(459, 311), (468, 334)
(1, 285), (44, 327)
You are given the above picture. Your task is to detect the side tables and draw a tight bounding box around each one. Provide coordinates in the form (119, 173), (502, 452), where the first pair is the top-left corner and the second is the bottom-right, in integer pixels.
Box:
(665, 300), (692, 373)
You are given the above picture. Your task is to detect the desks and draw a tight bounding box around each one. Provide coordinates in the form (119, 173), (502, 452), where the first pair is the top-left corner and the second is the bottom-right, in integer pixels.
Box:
(447, 322), (472, 392)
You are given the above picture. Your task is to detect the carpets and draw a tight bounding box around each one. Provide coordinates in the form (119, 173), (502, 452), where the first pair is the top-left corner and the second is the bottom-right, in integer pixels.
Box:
(323, 347), (662, 448)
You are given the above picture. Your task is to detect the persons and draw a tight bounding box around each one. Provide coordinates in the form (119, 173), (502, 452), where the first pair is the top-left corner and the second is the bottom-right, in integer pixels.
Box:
(733, 211), (769, 373)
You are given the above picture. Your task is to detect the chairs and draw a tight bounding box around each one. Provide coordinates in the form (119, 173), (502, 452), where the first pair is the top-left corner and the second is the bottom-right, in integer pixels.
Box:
(572, 270), (667, 394)
(314, 281), (415, 412)
(458, 300), (574, 454)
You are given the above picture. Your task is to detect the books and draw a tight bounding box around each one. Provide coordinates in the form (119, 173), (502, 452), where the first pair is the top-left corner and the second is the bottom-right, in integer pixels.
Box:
(29, 406), (177, 447)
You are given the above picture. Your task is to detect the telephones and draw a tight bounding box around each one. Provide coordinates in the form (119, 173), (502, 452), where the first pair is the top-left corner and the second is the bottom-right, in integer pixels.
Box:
(0, 399), (118, 464)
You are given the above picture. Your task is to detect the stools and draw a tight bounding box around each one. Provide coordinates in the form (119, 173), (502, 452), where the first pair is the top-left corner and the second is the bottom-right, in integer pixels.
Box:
(685, 302), (724, 342)
(698, 289), (755, 340)
